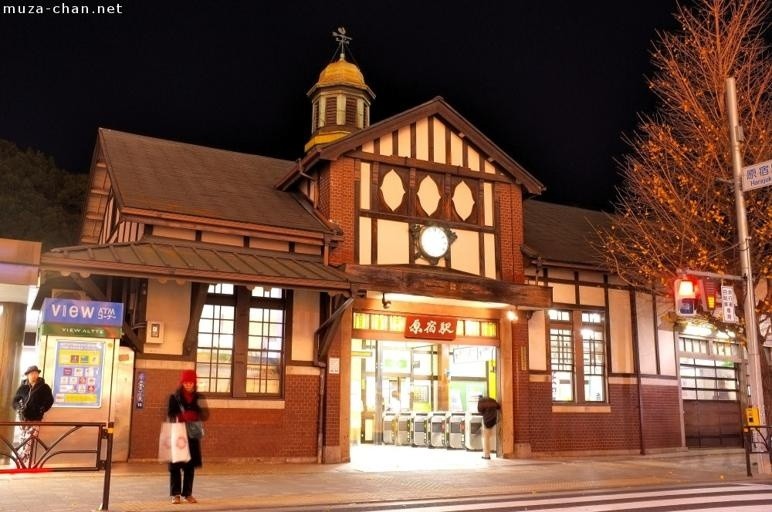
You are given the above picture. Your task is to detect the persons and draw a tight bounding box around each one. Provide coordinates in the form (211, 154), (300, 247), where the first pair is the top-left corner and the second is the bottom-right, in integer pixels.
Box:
(12, 366), (55, 468)
(164, 370), (211, 504)
(477, 394), (502, 459)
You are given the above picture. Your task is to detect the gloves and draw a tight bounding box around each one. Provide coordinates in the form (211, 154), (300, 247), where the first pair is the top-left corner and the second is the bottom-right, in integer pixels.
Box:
(182, 411), (199, 420)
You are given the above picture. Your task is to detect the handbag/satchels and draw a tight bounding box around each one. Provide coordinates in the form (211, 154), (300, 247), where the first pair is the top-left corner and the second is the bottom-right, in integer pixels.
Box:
(188, 423), (202, 439)
(158, 415), (192, 464)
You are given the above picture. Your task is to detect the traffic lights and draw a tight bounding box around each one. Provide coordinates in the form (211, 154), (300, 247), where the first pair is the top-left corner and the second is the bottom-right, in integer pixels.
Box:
(674, 269), (716, 318)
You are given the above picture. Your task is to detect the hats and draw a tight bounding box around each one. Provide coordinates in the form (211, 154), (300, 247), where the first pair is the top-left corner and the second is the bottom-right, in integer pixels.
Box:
(180, 370), (196, 382)
(25, 365), (41, 375)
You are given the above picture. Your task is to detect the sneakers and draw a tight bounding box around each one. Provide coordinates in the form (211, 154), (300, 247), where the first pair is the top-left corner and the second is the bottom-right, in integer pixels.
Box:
(172, 496), (180, 504)
(184, 496), (197, 503)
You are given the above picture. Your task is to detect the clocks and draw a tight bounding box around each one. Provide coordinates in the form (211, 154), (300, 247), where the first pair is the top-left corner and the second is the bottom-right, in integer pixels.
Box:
(411, 224), (458, 266)
